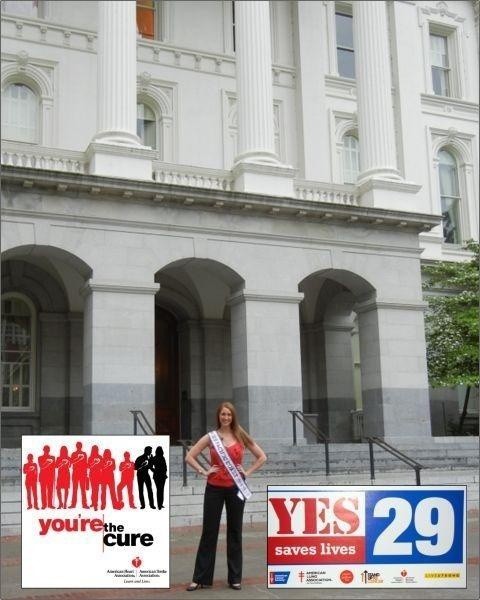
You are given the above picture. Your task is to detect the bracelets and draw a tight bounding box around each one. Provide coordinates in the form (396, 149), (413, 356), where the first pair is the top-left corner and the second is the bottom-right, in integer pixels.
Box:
(198, 467), (204, 474)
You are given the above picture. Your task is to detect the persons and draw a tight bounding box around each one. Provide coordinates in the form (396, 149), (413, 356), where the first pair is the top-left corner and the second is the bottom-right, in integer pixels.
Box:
(184, 402), (268, 591)
(23, 441), (167, 509)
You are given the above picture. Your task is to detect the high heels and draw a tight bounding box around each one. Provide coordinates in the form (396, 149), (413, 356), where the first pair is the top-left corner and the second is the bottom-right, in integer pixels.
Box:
(230, 584), (241, 590)
(186, 583), (204, 591)
